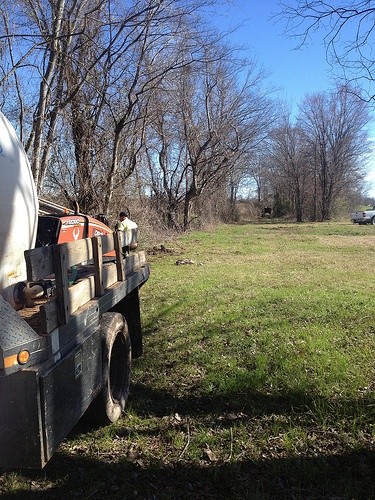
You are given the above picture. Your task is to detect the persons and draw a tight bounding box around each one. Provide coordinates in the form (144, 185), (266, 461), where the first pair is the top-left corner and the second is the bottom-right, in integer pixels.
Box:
(115, 211), (138, 259)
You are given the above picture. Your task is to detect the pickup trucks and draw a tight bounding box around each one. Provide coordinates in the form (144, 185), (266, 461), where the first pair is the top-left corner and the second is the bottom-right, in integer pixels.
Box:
(350, 206), (375, 226)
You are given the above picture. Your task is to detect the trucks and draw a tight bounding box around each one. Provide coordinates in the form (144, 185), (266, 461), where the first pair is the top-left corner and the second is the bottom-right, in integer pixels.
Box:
(0, 112), (151, 471)
